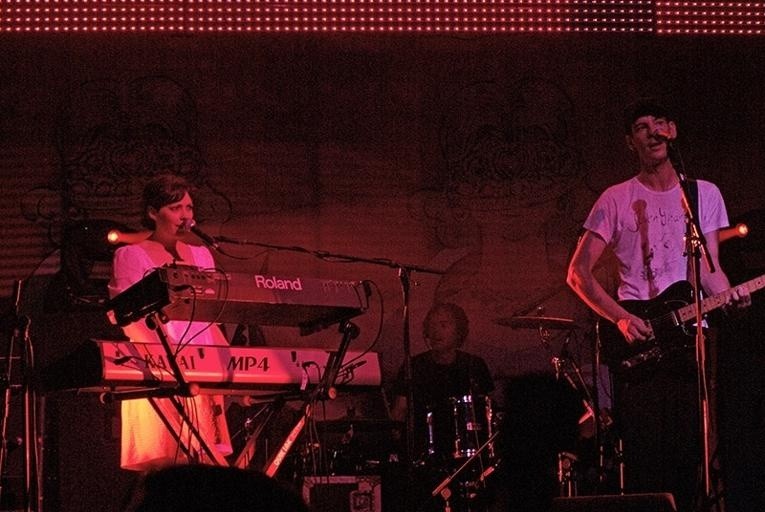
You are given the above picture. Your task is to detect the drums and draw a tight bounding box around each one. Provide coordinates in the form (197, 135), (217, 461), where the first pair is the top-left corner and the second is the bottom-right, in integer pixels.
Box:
(425, 395), (490, 470)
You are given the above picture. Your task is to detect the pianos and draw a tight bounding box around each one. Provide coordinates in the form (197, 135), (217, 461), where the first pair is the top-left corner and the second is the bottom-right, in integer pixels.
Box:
(40, 338), (384, 394)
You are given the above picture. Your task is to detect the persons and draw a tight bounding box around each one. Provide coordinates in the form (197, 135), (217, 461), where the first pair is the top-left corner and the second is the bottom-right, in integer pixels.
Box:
(389, 301), (499, 461)
(104, 172), (234, 476)
(562, 100), (753, 510)
(37, 212), (106, 511)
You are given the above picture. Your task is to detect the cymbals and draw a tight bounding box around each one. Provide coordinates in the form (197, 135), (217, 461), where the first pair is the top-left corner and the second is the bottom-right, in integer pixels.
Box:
(497, 317), (578, 332)
(316, 417), (406, 434)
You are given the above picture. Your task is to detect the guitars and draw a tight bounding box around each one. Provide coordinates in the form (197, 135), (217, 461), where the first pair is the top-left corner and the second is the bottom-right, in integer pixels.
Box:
(599, 275), (764, 376)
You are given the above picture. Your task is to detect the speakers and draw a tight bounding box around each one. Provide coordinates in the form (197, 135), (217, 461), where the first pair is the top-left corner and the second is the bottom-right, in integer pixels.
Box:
(552, 492), (678, 512)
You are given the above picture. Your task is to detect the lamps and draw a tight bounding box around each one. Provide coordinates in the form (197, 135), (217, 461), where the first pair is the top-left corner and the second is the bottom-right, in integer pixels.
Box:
(64, 219), (138, 266)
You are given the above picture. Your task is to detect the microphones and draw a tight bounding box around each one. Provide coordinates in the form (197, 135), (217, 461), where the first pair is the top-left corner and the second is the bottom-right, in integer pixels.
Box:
(184, 219), (224, 255)
(649, 126), (676, 143)
(422, 330), (431, 351)
